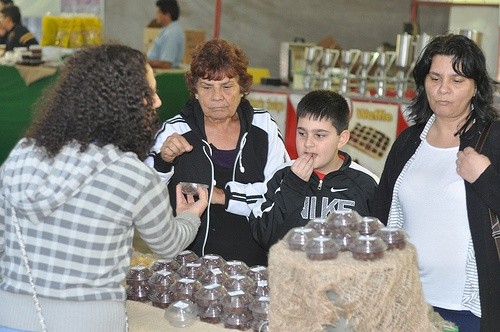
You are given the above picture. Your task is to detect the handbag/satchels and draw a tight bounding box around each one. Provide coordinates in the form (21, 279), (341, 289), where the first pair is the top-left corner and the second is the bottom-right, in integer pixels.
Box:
(473, 117), (499, 265)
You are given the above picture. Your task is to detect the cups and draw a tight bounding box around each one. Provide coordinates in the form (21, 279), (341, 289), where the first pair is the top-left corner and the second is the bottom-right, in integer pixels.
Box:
(302, 28), (485, 99)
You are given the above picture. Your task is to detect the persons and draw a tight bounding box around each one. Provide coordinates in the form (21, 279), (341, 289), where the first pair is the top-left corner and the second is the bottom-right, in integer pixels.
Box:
(0, 44), (209, 332)
(146, 0), (186, 70)
(145, 39), (291, 268)
(249, 91), (380, 257)
(0, 0), (40, 49)
(374, 34), (500, 332)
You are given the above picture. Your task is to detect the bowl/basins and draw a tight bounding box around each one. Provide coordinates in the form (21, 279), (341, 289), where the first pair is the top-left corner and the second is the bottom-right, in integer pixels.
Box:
(180, 182), (209, 196)
(126, 250), (273, 332)
(5, 44), (42, 66)
(281, 208), (406, 262)
